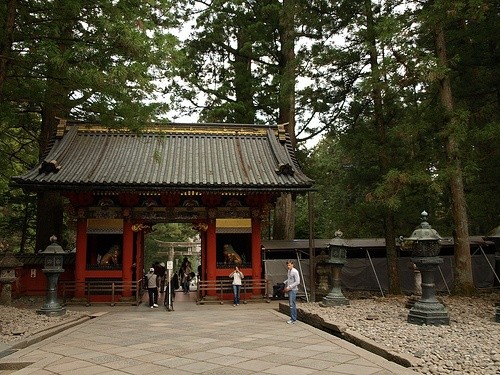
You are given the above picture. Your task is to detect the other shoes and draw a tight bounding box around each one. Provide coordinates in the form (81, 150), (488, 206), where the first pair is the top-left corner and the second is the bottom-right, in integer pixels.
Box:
(233, 304), (236, 306)
(237, 303), (239, 305)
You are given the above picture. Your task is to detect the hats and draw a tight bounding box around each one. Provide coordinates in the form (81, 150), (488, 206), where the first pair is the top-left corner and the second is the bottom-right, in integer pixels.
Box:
(150, 268), (155, 271)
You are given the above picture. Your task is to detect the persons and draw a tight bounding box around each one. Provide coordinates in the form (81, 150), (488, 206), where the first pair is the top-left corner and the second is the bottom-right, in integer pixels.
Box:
(144, 257), (192, 311)
(229, 266), (244, 307)
(284, 260), (300, 324)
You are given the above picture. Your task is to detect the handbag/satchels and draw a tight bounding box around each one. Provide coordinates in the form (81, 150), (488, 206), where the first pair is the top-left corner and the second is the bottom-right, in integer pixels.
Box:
(145, 280), (148, 286)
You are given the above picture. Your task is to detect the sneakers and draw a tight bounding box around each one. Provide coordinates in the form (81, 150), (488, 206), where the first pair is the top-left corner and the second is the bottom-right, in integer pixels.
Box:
(153, 303), (159, 308)
(286, 319), (296, 324)
(150, 306), (153, 308)
